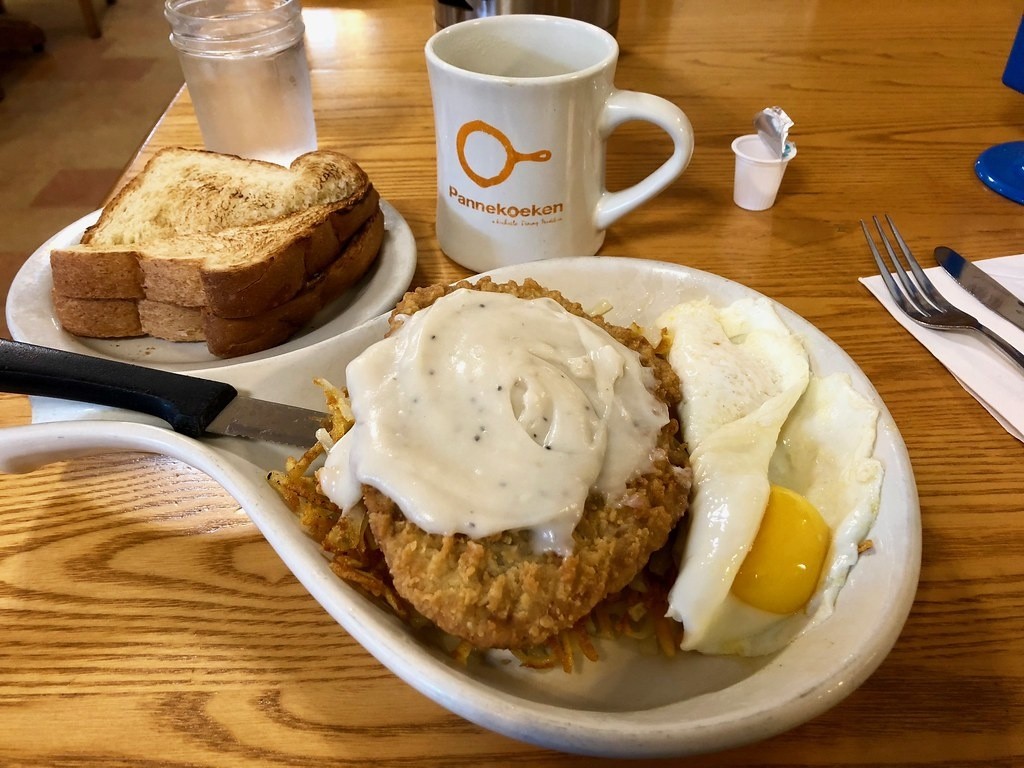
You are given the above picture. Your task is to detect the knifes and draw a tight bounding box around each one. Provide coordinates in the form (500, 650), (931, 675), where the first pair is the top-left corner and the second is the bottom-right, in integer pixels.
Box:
(0, 340), (353, 449)
(933, 246), (1024, 333)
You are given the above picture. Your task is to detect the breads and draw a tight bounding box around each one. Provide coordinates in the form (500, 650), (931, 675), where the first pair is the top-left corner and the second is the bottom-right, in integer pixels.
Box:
(51, 206), (384, 358)
(51, 143), (381, 320)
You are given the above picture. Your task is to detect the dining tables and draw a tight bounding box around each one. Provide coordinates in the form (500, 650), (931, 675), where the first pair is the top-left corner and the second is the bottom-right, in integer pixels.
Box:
(0, 0), (1024, 768)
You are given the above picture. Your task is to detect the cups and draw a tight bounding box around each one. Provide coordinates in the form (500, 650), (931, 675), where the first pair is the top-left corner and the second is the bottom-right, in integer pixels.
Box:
(162, 0), (318, 167)
(425, 14), (694, 273)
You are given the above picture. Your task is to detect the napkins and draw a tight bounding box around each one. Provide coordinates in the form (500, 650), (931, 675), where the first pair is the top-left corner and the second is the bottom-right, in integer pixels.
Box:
(857, 253), (1024, 444)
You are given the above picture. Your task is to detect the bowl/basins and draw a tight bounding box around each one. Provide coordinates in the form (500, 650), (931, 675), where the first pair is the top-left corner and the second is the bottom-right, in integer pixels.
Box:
(0, 253), (924, 755)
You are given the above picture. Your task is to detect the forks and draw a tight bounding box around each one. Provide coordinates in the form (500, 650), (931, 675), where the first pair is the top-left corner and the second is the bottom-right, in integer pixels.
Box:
(861, 214), (1024, 374)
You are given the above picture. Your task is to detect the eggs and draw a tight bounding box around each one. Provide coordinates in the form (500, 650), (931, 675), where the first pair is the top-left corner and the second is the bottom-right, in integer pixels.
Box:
(656, 294), (885, 657)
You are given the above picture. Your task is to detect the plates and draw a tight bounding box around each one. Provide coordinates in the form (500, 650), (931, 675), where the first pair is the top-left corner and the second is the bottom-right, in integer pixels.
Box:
(5, 196), (418, 369)
